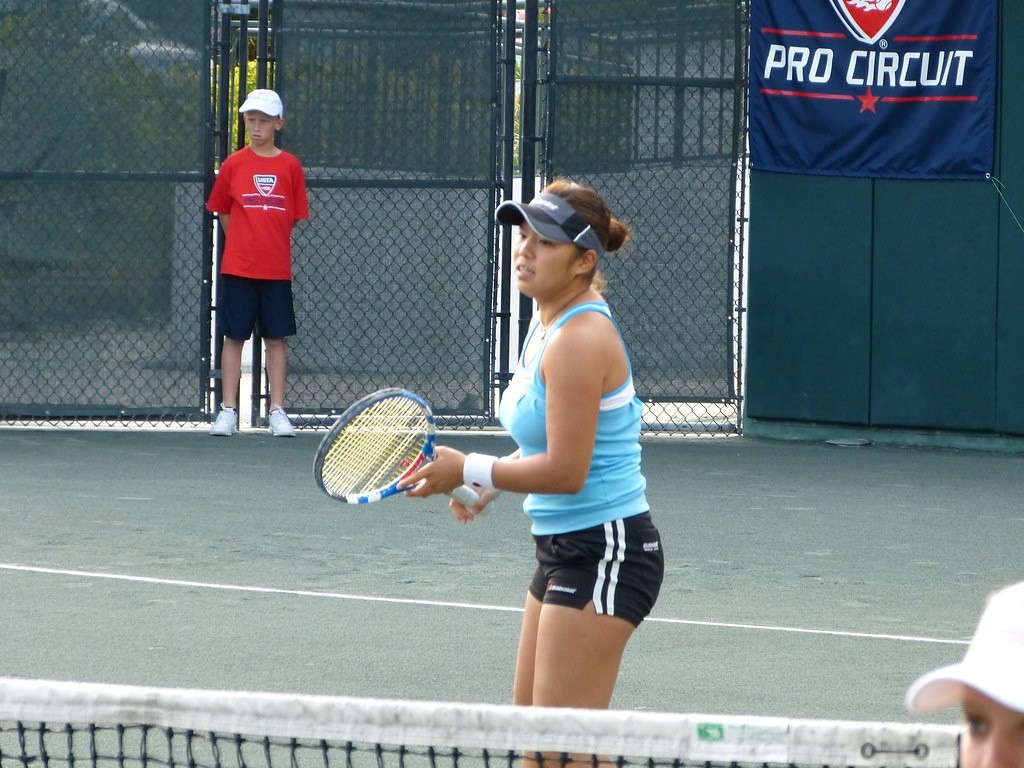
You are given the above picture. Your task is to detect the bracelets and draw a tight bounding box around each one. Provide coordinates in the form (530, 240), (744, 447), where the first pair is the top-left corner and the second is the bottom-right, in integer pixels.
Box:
(463, 452), (500, 495)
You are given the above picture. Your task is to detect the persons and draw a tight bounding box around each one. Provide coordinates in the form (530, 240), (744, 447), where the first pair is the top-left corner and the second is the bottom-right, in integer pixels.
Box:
(905, 580), (1024, 768)
(396, 178), (665, 768)
(205, 88), (309, 436)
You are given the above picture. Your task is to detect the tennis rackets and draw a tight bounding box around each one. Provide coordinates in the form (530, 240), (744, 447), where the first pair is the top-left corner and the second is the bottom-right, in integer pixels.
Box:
(312, 386), (482, 513)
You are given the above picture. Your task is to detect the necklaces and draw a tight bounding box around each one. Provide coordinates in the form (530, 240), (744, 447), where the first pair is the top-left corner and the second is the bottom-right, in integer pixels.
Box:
(538, 300), (563, 340)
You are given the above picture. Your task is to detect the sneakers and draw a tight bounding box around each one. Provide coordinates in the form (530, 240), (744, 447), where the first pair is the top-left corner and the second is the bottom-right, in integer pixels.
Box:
(267, 405), (296, 437)
(209, 403), (238, 436)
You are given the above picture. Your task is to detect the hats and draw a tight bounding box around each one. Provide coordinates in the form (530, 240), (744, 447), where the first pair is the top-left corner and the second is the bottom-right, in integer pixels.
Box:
(904, 582), (1024, 714)
(494, 191), (605, 257)
(238, 88), (284, 119)
